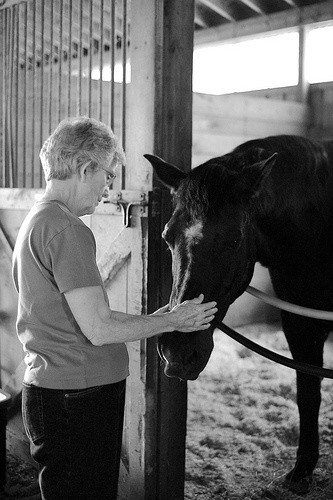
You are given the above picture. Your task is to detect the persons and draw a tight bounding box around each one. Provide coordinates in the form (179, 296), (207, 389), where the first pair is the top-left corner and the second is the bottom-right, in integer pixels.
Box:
(12, 117), (219, 500)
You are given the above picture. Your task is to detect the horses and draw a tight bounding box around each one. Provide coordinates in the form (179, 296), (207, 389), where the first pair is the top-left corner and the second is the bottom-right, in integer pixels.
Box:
(144, 134), (333, 491)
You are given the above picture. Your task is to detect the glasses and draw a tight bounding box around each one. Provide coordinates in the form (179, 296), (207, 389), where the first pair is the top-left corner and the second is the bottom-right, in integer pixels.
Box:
(99, 167), (117, 186)
